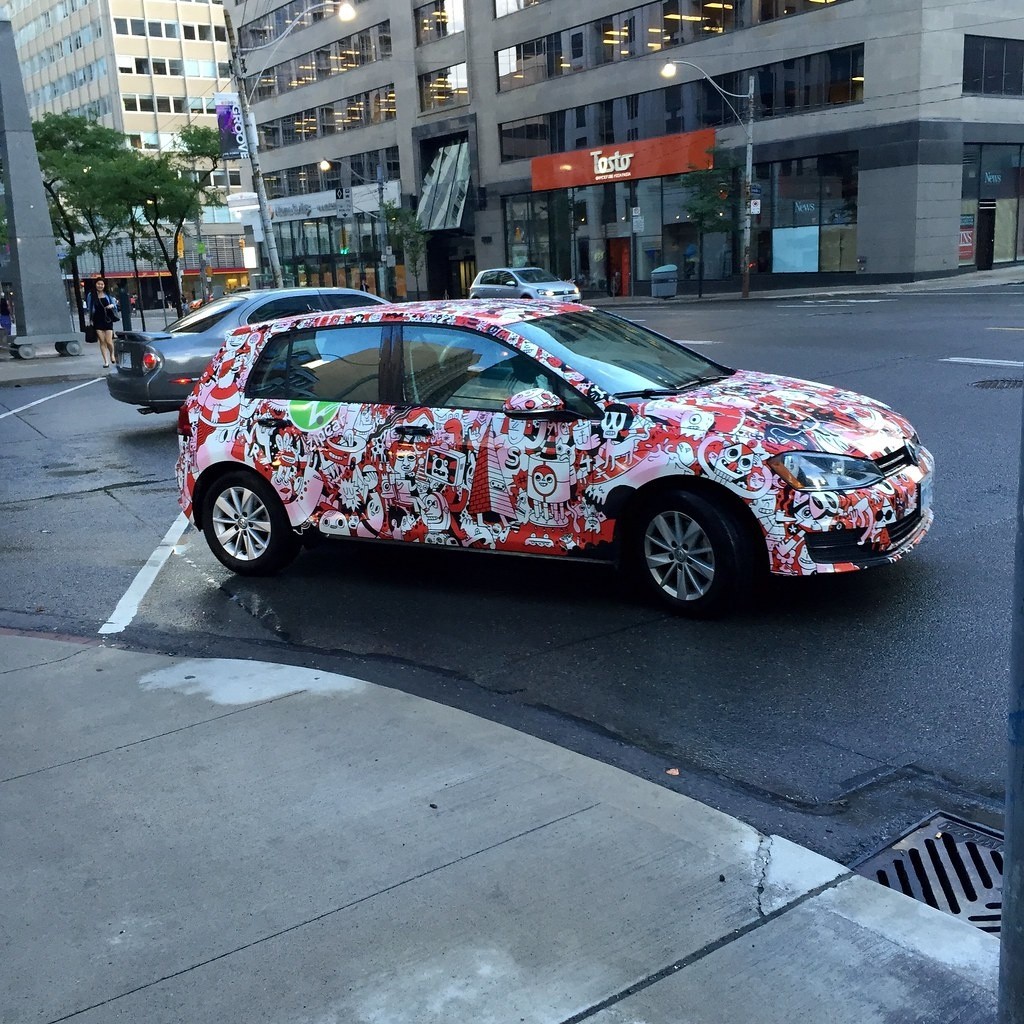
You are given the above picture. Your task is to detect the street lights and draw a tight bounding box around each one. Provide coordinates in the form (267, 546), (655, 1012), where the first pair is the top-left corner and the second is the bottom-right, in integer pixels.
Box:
(659, 60), (755, 297)
(223, 0), (357, 287)
(319, 157), (392, 297)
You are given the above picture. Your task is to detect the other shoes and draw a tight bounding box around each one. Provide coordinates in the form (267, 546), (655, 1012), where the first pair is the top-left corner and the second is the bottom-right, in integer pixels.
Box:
(103, 364), (110, 368)
(111, 360), (116, 363)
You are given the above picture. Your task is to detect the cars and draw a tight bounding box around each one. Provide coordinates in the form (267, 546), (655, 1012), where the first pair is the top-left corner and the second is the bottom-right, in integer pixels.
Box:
(106, 287), (392, 416)
(174, 298), (937, 607)
(470, 266), (581, 305)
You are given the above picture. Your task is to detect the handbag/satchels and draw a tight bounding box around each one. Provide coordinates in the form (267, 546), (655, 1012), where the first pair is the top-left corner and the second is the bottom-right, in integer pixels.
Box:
(84, 324), (98, 343)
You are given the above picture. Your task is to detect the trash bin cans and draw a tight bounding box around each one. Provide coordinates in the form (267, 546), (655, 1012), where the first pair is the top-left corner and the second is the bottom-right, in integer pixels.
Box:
(651, 264), (678, 297)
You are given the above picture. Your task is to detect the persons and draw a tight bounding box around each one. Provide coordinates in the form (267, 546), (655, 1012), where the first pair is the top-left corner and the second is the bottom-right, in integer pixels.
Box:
(87, 277), (120, 368)
(181, 295), (187, 312)
(130, 296), (136, 311)
(114, 297), (119, 312)
(82, 300), (87, 315)
(360, 279), (369, 292)
(0, 293), (14, 335)
